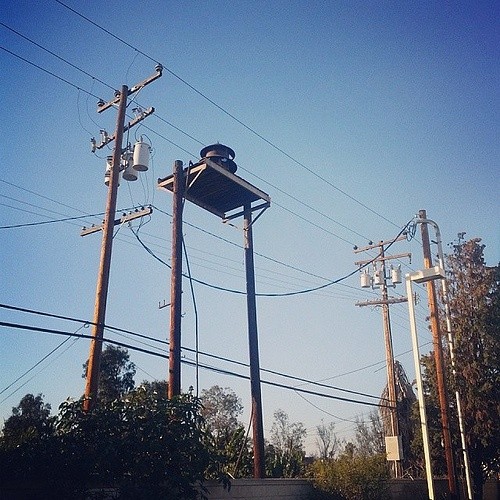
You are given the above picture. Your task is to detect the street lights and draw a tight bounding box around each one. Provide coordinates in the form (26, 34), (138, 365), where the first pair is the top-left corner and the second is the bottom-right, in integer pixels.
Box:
(412, 217), (473, 500)
(404, 267), (458, 500)
